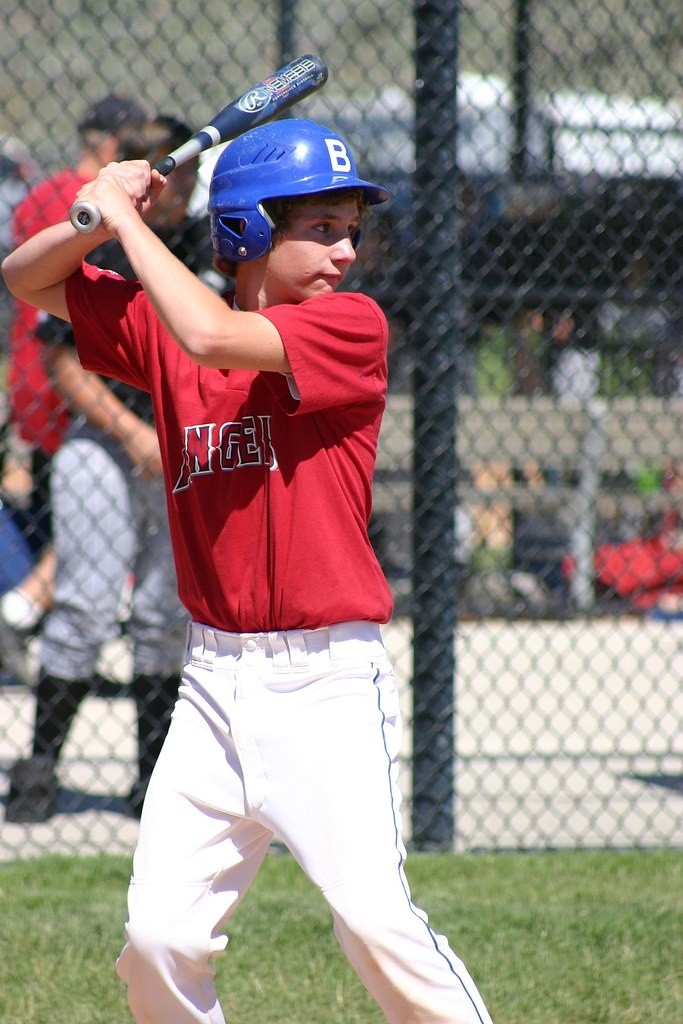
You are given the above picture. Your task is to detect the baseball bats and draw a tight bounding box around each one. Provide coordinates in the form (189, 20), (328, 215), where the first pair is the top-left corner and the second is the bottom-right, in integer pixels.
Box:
(71, 51), (327, 234)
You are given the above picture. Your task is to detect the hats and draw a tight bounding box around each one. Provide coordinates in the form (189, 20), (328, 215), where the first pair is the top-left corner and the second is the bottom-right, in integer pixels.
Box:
(78, 95), (148, 132)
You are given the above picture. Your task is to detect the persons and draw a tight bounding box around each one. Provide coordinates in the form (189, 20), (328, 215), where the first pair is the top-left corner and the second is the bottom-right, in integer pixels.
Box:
(0, 119), (495, 1023)
(0, 100), (244, 824)
(452, 312), (682, 615)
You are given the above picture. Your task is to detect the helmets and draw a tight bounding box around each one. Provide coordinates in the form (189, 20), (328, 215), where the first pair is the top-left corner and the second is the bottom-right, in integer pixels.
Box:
(205, 119), (391, 262)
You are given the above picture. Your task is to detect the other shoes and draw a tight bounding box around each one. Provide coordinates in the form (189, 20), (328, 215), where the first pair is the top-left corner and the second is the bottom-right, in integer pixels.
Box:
(7, 759), (63, 822)
